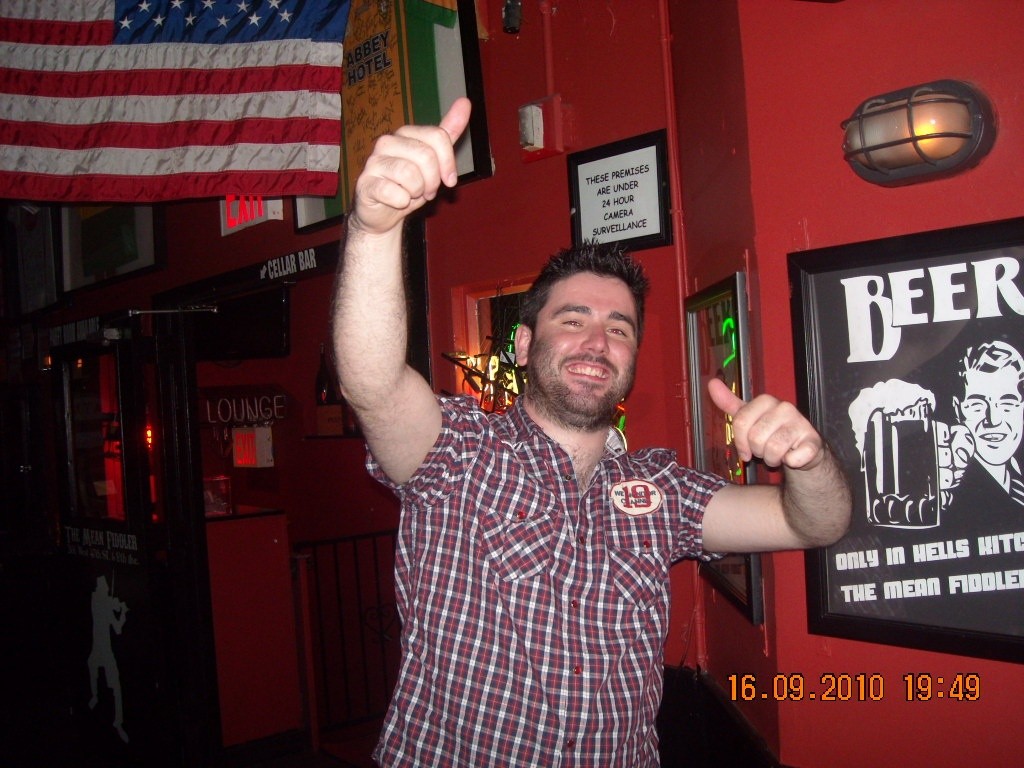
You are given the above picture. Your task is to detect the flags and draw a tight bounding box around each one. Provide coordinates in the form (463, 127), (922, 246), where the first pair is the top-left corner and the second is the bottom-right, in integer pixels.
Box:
(0, 0), (354, 202)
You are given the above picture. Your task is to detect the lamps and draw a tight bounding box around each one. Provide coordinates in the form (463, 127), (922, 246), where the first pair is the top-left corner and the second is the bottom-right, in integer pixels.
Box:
(840, 78), (999, 187)
(442, 285), (527, 416)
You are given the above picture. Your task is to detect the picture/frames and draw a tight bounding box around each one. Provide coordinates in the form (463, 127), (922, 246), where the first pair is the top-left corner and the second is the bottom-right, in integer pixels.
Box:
(8, 203), (61, 318)
(683, 272), (765, 626)
(787, 217), (1024, 664)
(56, 203), (167, 301)
(566, 128), (674, 254)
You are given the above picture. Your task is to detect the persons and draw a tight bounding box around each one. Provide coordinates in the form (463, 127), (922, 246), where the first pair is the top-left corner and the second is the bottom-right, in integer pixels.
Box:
(333, 99), (851, 768)
